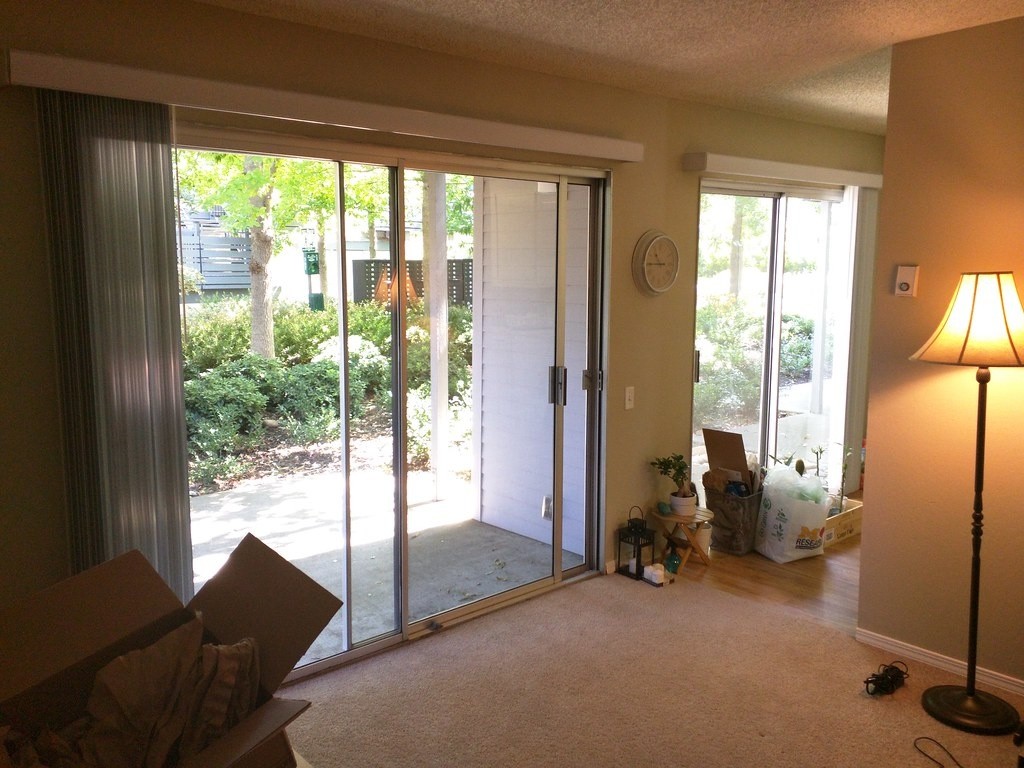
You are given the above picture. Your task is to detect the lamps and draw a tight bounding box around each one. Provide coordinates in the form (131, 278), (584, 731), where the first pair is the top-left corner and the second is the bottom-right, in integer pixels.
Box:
(617, 506), (656, 580)
(909, 270), (1023, 740)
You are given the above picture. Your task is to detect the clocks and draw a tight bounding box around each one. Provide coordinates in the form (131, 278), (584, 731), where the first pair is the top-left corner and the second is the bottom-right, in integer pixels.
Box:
(631, 229), (680, 297)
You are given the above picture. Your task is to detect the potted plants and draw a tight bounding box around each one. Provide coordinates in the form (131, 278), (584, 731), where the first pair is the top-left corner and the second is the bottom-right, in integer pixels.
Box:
(652, 452), (697, 516)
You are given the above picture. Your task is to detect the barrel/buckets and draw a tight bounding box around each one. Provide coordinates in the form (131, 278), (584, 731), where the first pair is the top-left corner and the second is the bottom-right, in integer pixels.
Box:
(689, 524), (712, 554)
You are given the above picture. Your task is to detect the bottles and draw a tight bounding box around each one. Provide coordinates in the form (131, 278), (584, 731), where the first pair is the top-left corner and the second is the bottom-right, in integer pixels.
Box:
(664, 542), (680, 573)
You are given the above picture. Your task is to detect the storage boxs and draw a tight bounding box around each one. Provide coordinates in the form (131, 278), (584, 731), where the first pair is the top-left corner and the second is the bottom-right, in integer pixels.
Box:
(824, 499), (864, 549)
(1, 531), (343, 768)
(705, 488), (769, 556)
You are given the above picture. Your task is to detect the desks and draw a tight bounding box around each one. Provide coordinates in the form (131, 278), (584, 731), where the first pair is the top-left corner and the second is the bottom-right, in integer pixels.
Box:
(653, 507), (715, 575)
